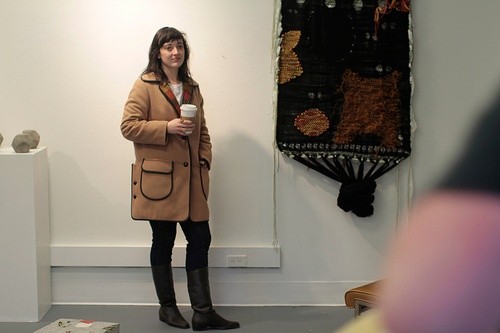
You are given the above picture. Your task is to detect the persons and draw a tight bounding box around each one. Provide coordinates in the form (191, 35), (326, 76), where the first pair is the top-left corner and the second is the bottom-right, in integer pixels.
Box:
(121, 27), (241, 331)
(334, 83), (500, 333)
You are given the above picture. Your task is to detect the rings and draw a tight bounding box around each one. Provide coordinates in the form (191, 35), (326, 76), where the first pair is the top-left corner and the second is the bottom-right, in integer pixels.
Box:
(182, 130), (185, 133)
(181, 119), (184, 123)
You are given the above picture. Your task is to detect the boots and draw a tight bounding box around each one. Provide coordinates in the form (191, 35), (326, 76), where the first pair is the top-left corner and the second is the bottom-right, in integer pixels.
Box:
(152, 264), (190, 329)
(187, 266), (240, 331)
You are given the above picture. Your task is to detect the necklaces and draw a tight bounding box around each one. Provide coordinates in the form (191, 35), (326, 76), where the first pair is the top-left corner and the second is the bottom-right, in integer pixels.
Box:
(168, 81), (180, 96)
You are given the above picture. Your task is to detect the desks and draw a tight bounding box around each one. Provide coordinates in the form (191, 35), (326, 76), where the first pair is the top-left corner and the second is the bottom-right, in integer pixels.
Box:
(0, 146), (52, 322)
(344, 277), (389, 323)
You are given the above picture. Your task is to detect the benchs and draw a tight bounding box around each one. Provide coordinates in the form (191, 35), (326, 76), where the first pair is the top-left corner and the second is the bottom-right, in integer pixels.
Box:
(34, 318), (121, 333)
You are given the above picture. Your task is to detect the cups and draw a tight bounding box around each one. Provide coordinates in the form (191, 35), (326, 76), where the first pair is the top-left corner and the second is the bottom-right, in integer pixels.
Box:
(179, 103), (197, 135)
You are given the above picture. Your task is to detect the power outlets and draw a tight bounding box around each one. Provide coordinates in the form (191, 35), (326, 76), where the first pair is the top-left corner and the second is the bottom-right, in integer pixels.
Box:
(226, 254), (247, 268)
